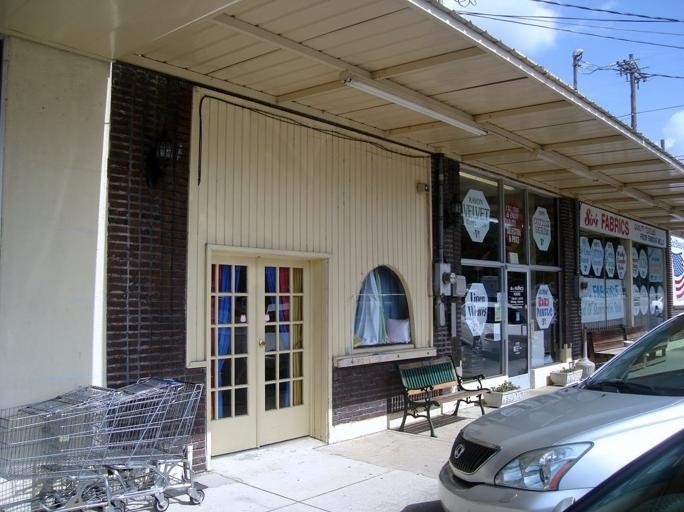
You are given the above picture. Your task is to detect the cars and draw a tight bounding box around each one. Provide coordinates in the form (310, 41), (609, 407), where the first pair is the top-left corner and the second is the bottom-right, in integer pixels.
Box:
(461, 302), (530, 358)
(651, 297), (666, 316)
(438, 310), (683, 512)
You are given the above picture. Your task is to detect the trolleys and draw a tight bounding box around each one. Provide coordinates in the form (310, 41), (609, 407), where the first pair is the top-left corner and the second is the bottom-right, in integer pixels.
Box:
(0, 369), (206, 512)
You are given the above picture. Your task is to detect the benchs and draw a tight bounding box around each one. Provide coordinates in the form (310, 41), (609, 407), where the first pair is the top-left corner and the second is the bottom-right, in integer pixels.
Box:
(623, 325), (668, 367)
(396, 353), (490, 439)
(586, 328), (634, 374)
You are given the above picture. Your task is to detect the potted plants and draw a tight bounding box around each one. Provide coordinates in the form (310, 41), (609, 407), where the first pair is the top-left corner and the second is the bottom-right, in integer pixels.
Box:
(548, 364), (584, 388)
(482, 384), (524, 410)
